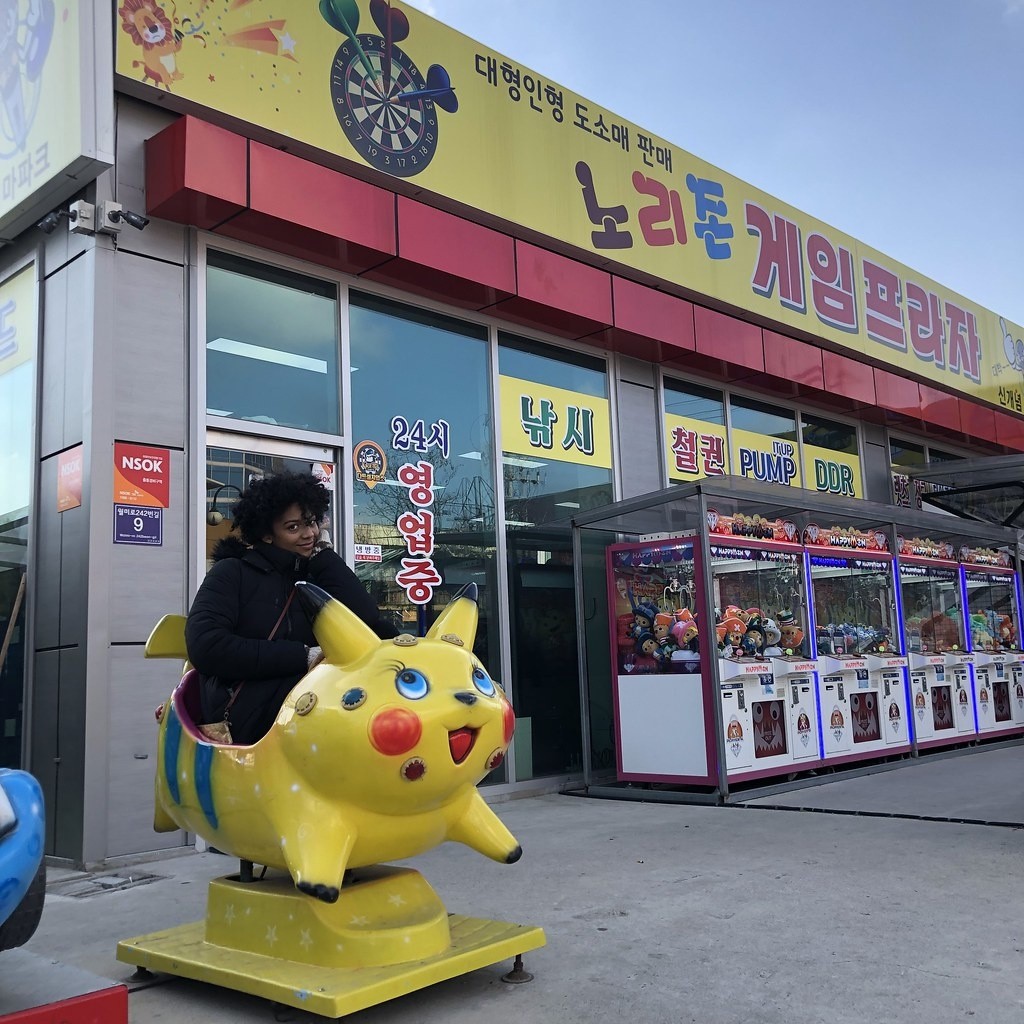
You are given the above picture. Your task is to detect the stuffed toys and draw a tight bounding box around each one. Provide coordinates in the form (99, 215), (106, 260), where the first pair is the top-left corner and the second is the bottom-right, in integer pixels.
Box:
(617, 602), (1019, 670)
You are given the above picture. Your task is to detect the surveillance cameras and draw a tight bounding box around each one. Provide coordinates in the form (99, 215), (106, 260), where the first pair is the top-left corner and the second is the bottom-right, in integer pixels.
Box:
(38, 212), (61, 234)
(124, 210), (150, 231)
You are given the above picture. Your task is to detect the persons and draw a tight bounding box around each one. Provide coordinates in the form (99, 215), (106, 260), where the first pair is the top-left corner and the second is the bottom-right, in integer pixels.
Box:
(185, 473), (399, 745)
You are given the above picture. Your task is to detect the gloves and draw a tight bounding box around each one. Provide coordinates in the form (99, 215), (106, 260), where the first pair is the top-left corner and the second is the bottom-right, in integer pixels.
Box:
(309, 529), (333, 561)
(305, 646), (326, 668)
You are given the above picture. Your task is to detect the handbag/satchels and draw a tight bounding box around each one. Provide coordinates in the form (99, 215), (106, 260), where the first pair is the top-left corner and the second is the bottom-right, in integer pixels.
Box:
(196, 721), (233, 745)
